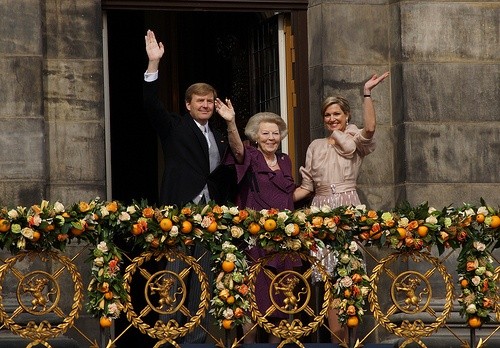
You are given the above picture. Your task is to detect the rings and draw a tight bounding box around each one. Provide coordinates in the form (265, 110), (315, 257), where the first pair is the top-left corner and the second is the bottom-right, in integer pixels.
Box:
(380, 80), (383, 83)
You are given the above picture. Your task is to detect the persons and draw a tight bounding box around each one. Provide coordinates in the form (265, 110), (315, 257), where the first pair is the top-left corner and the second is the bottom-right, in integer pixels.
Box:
(141, 29), (237, 348)
(295, 71), (390, 342)
(215, 95), (304, 343)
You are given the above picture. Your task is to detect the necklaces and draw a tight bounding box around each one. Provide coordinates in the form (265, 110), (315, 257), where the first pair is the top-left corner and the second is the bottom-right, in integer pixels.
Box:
(258, 148), (277, 167)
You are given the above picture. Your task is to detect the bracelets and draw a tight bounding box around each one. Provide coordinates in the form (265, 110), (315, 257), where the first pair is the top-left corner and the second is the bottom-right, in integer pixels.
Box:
(363, 94), (371, 97)
(227, 126), (237, 132)
